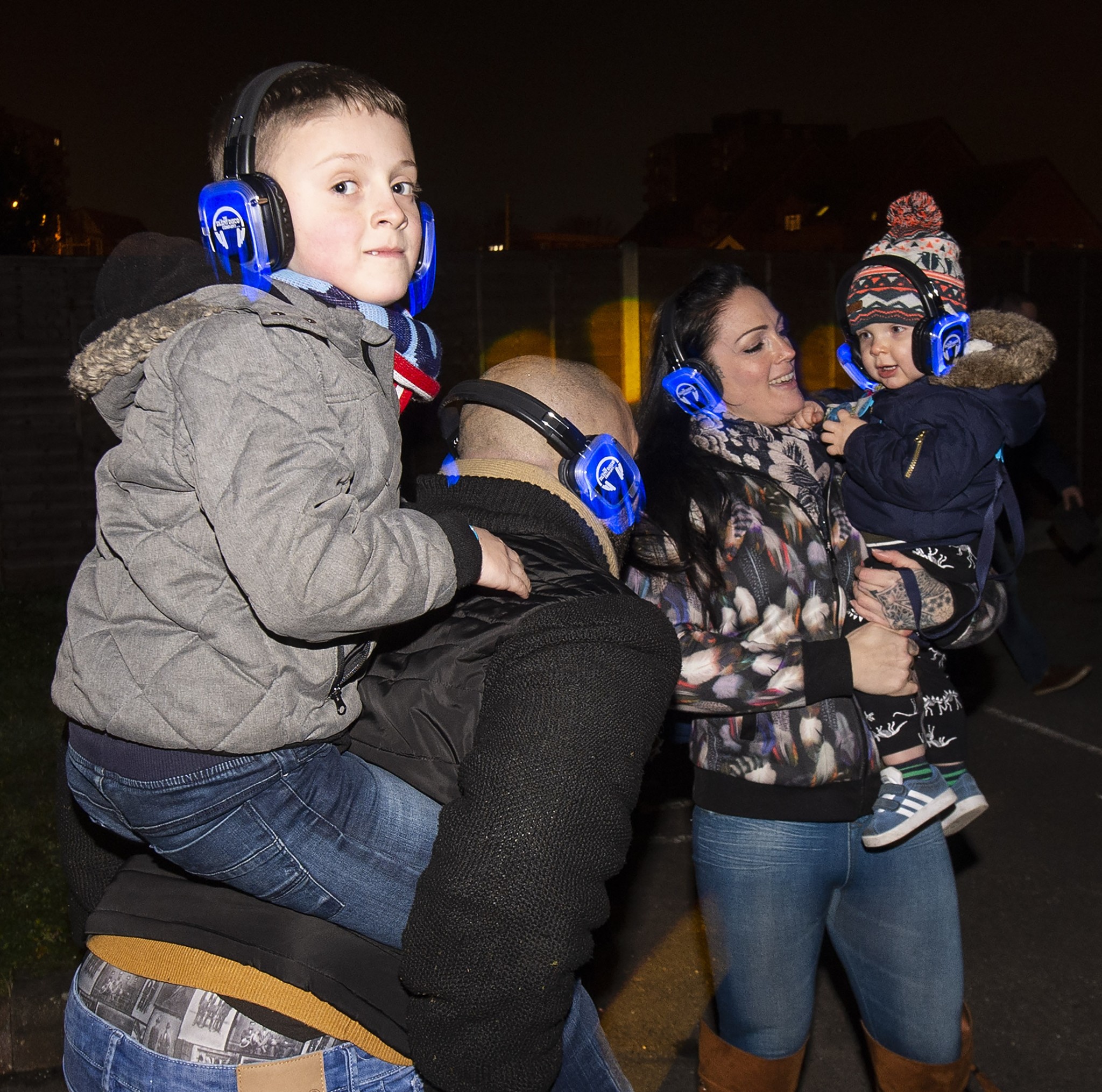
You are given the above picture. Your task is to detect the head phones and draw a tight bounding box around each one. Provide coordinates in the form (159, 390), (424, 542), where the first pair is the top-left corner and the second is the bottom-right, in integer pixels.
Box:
(197, 61), (441, 321)
(436, 378), (646, 538)
(834, 254), (972, 393)
(661, 297), (726, 420)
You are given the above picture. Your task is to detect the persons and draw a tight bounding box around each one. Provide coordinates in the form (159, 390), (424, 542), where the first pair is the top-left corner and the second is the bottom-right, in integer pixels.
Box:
(62, 356), (681, 1090)
(51, 59), (637, 1092)
(625, 254), (979, 1092)
(789, 190), (1058, 849)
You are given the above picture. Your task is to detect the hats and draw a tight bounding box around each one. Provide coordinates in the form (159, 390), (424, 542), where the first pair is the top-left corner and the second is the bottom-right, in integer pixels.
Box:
(845, 191), (968, 330)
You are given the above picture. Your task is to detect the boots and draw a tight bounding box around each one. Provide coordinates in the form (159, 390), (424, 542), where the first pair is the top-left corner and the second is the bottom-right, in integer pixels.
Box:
(696, 1022), (809, 1092)
(860, 1004), (974, 1092)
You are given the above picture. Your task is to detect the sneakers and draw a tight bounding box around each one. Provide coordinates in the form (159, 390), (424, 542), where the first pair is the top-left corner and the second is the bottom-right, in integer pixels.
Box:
(940, 771), (990, 836)
(862, 763), (958, 849)
